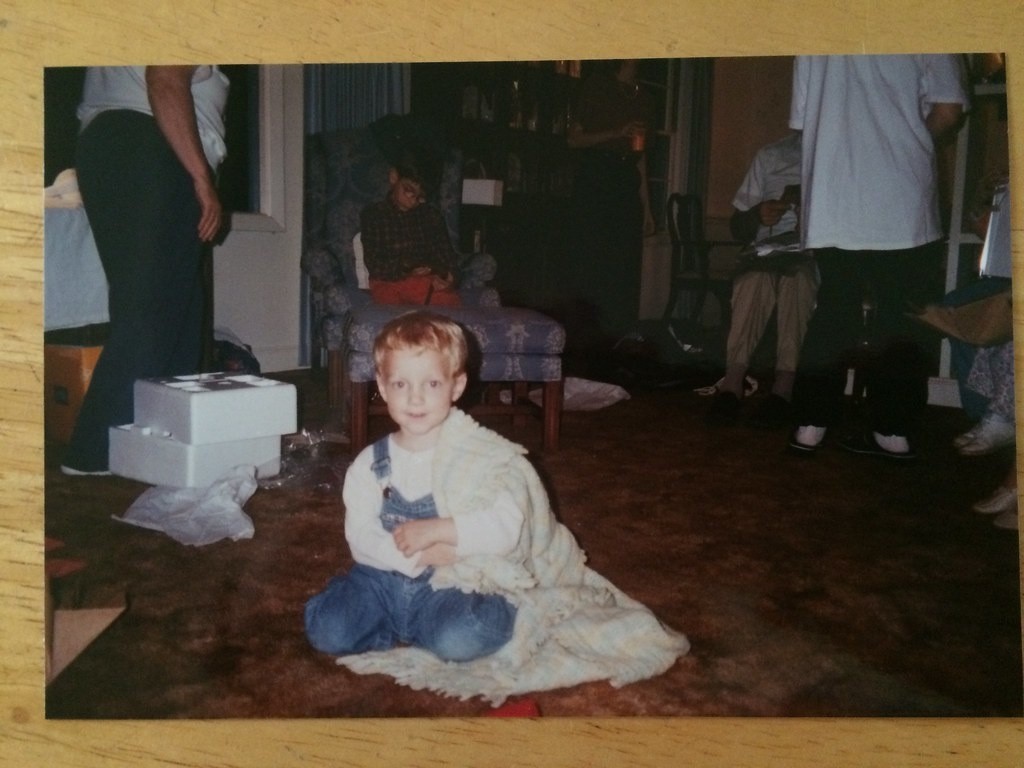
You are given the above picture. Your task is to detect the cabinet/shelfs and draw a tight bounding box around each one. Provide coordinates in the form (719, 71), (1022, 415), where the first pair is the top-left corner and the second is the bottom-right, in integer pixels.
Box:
(932, 81), (1006, 382)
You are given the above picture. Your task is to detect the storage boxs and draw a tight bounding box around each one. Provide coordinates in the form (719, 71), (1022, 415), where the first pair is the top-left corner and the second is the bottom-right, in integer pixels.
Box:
(108, 373), (299, 489)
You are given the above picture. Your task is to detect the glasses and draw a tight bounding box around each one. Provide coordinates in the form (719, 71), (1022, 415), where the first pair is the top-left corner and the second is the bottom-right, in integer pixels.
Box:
(398, 175), (429, 204)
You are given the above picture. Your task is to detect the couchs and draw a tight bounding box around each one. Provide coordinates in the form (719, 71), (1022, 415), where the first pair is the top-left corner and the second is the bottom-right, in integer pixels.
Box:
(300, 113), (498, 410)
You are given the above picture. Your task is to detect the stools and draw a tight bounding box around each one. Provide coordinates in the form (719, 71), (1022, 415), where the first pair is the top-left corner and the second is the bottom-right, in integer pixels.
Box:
(345, 301), (567, 457)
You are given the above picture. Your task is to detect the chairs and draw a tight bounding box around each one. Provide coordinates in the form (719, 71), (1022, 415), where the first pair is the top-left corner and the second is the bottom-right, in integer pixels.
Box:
(659, 192), (743, 348)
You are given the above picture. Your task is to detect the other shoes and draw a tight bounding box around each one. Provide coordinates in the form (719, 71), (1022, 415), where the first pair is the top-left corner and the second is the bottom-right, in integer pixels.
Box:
(752, 395), (792, 432)
(971, 486), (1017, 514)
(60, 445), (113, 477)
(708, 392), (736, 426)
(953, 416), (1015, 458)
(993, 511), (1018, 531)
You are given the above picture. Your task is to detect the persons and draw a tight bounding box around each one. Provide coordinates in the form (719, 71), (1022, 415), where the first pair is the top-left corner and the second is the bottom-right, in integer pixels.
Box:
(702, 129), (823, 431)
(788, 55), (973, 461)
(300, 309), (533, 663)
(356, 148), (465, 309)
(59, 64), (231, 477)
(949, 52), (1018, 527)
(566, 62), (659, 362)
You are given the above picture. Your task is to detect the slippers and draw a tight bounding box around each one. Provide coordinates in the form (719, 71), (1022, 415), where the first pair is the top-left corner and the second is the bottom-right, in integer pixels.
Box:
(848, 430), (919, 464)
(787, 424), (819, 459)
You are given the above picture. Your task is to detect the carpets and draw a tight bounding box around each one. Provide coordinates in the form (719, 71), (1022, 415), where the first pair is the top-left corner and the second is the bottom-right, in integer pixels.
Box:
(44, 374), (1023, 717)
